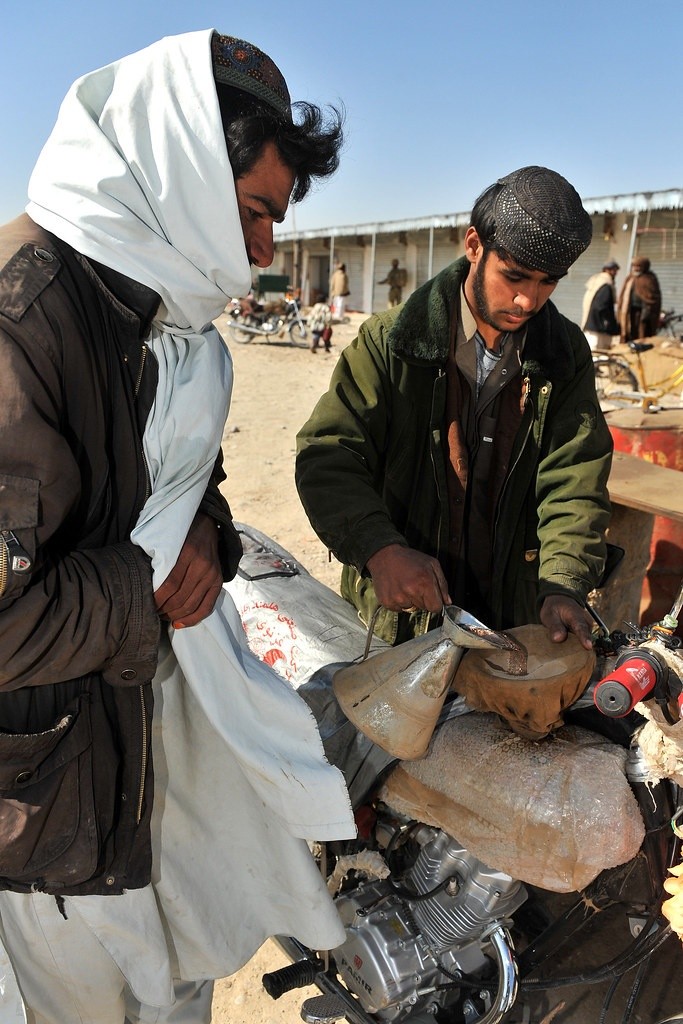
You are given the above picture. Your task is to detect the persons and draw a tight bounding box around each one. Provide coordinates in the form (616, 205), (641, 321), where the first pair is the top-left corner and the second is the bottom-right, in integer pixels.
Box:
(614, 256), (660, 345)
(327, 261), (351, 320)
(375, 258), (403, 311)
(304, 294), (331, 354)
(578, 262), (622, 356)
(293, 166), (624, 659)
(0, 28), (361, 1021)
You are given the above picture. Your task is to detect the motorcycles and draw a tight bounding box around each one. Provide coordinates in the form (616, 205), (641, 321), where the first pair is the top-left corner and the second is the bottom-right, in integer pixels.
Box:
(216, 512), (683, 1024)
(226, 291), (316, 349)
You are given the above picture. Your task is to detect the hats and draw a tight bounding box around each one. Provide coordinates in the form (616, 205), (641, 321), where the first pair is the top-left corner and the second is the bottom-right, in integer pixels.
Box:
(210, 33), (294, 119)
(493, 166), (595, 274)
(603, 261), (622, 269)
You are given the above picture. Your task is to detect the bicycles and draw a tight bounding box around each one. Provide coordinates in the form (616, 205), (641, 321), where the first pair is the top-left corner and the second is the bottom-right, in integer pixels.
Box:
(589, 333), (683, 421)
